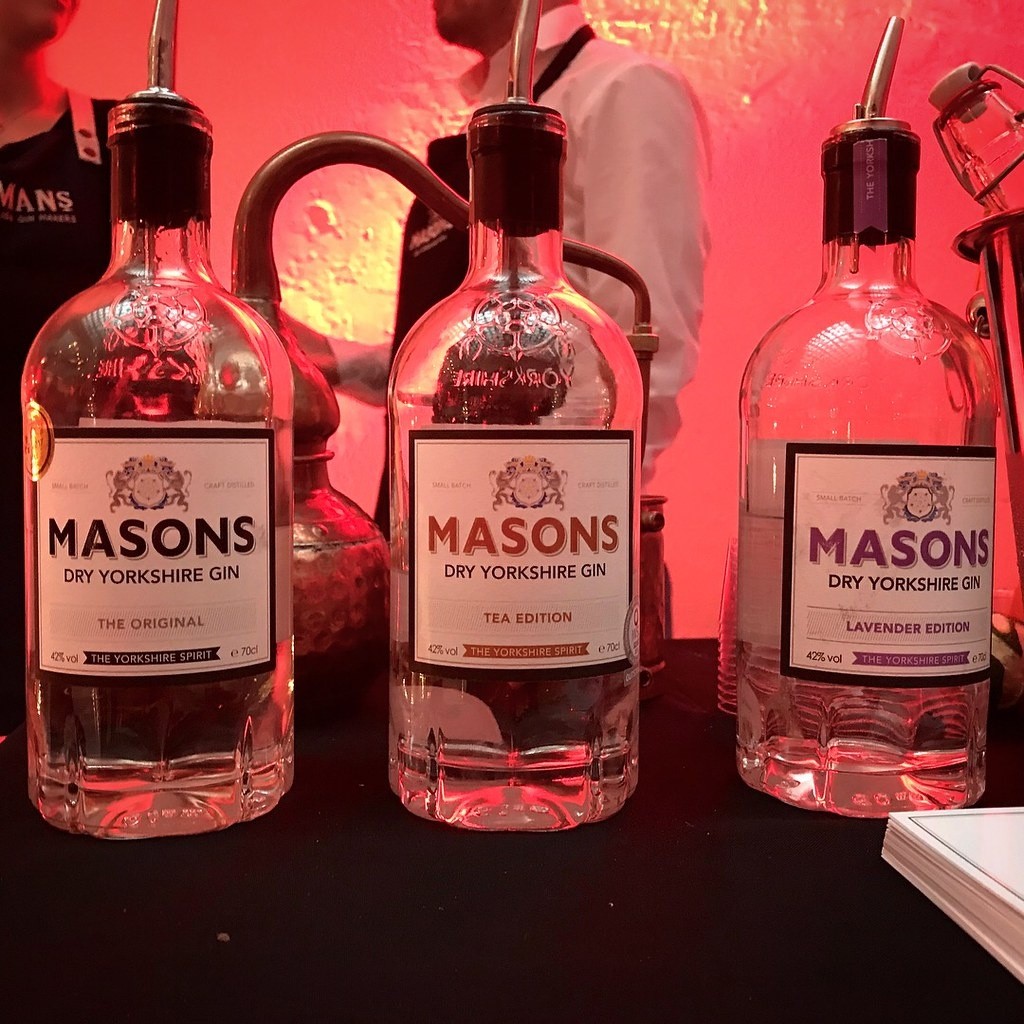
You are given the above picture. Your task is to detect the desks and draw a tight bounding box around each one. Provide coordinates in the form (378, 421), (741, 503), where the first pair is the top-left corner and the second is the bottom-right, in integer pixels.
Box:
(0, 636), (1024, 1024)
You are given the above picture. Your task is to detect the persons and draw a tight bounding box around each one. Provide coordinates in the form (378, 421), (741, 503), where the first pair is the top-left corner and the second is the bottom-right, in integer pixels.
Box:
(279, 1), (710, 539)
(0, 0), (123, 733)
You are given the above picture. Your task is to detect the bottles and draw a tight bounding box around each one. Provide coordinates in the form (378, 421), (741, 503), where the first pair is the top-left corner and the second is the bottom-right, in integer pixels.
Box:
(385, 1), (646, 833)
(22, 0), (295, 842)
(735, 12), (997, 817)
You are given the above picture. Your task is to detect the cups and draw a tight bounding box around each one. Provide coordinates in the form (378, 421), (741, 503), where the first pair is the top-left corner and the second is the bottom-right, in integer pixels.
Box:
(718, 539), (739, 715)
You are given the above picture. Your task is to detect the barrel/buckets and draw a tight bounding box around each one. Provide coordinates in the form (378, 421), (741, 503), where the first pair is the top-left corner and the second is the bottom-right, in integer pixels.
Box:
(952, 205), (1024, 623)
